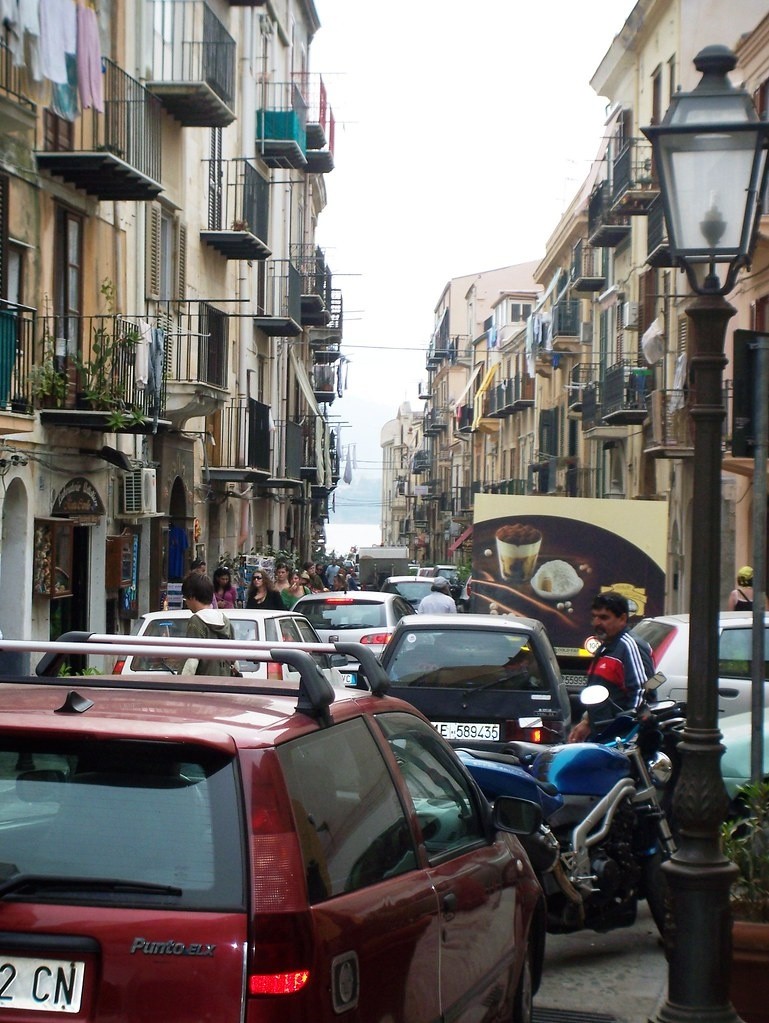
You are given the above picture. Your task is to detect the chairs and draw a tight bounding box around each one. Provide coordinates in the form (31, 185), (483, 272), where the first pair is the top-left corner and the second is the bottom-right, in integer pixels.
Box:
(32, 771), (205, 889)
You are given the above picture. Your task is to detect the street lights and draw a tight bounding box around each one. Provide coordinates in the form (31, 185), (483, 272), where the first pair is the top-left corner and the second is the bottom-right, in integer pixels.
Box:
(635, 40), (769, 1021)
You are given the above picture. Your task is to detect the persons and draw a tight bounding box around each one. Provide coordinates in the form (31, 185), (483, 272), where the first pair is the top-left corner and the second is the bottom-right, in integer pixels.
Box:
(728, 566), (769, 611)
(177, 571), (237, 676)
(418, 576), (457, 613)
(568, 591), (658, 744)
(329, 550), (336, 558)
(431, 566), (439, 577)
(246, 559), (363, 611)
(191, 557), (238, 609)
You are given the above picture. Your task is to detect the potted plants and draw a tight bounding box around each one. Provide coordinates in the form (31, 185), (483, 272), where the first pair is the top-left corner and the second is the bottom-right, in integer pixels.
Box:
(28, 337), (70, 409)
(70, 327), (146, 433)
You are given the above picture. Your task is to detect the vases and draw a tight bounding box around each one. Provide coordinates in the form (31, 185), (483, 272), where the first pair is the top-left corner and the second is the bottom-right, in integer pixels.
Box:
(11, 399), (28, 414)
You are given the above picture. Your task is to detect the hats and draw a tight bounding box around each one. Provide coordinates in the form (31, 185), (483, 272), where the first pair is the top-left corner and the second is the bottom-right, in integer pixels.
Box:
(737, 566), (754, 580)
(433, 576), (448, 588)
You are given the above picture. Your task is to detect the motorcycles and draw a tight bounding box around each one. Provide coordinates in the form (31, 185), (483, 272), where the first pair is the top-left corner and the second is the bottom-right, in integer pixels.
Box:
(430, 671), (688, 993)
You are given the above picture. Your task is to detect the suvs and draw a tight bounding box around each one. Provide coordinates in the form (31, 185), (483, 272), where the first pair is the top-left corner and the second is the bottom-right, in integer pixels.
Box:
(108, 541), (769, 810)
(0, 634), (546, 1022)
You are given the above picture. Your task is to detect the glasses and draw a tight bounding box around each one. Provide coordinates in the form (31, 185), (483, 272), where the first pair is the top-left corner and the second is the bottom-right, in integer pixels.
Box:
(290, 569), (299, 573)
(253, 576), (263, 580)
(594, 593), (615, 605)
(316, 568), (323, 572)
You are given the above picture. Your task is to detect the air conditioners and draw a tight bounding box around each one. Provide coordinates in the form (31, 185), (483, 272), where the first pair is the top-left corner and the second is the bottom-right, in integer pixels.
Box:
(123, 468), (157, 514)
(623, 301), (639, 331)
